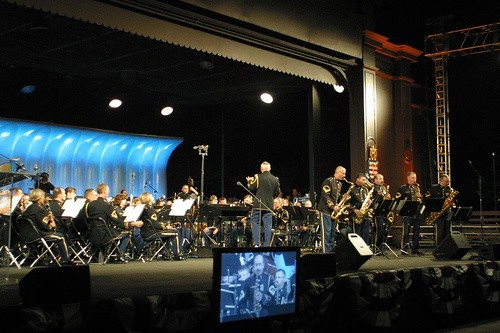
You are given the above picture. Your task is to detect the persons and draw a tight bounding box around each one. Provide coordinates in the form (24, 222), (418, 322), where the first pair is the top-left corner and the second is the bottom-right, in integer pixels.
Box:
(237, 266), (251, 283)
(317, 166), (348, 253)
(273, 269), (294, 306)
(397, 171), (424, 256)
(244, 161), (282, 247)
(424, 174), (457, 247)
(371, 175), (394, 255)
(237, 254), (277, 316)
(140, 184), (253, 259)
(351, 173), (372, 248)
(0, 183), (143, 265)
(274, 187), (317, 250)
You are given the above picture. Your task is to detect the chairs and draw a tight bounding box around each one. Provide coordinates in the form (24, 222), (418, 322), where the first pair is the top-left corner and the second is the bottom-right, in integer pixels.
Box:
(0, 205), (322, 270)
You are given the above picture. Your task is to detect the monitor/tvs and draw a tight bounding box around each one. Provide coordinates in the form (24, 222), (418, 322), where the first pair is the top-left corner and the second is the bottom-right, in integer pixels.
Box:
(213, 246), (300, 327)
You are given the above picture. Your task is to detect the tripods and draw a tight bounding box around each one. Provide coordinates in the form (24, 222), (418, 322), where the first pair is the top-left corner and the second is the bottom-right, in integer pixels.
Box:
(394, 201), (420, 257)
(370, 199), (399, 259)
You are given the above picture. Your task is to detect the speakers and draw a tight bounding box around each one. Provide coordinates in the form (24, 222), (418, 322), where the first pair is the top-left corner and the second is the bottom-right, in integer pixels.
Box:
(20, 265), (91, 306)
(432, 231), (471, 262)
(330, 233), (374, 272)
(478, 244), (500, 261)
(298, 253), (336, 278)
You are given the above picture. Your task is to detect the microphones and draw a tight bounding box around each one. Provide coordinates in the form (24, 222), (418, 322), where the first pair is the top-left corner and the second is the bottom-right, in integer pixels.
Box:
(5, 157), (22, 163)
(237, 181), (243, 187)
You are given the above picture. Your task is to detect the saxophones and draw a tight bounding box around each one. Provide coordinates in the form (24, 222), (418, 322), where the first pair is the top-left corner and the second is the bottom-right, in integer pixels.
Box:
(355, 183), (375, 224)
(425, 185), (459, 225)
(331, 178), (354, 220)
